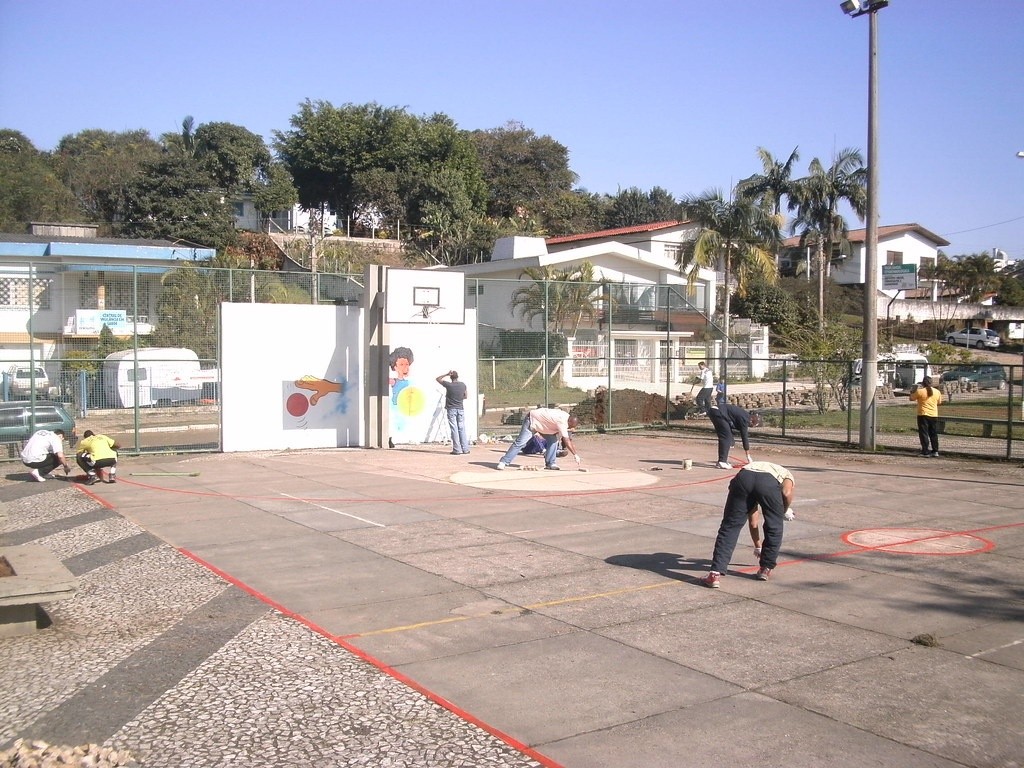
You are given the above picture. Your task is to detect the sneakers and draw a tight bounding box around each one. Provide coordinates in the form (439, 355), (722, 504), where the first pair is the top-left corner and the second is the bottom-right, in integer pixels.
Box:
(108, 473), (116, 482)
(695, 571), (720, 587)
(30, 469), (45, 482)
(497, 462), (505, 469)
(715, 461), (733, 469)
(43, 473), (55, 478)
(84, 475), (100, 485)
(756, 567), (770, 580)
(547, 464), (560, 470)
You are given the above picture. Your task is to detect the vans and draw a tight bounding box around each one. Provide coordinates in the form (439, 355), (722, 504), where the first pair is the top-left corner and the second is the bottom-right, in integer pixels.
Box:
(854, 354), (931, 391)
(7, 365), (49, 401)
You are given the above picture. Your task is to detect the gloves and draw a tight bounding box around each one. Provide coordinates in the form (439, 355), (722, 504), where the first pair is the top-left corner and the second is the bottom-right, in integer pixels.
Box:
(753, 547), (762, 559)
(573, 454), (581, 467)
(783, 508), (795, 521)
(746, 454), (753, 464)
(64, 464), (71, 475)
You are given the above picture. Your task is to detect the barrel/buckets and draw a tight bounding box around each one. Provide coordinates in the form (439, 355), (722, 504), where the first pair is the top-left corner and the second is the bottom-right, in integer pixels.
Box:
(682, 458), (692, 471)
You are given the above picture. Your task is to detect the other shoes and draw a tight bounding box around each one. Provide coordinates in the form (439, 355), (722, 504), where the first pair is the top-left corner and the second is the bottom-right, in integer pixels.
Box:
(450, 451), (460, 455)
(543, 451), (547, 457)
(918, 454), (930, 457)
(930, 452), (939, 457)
(556, 450), (568, 456)
(698, 412), (706, 416)
(463, 451), (470, 454)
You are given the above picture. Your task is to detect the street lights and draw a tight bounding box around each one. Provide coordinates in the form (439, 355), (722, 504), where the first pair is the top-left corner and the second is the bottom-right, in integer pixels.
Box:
(840, 0), (888, 450)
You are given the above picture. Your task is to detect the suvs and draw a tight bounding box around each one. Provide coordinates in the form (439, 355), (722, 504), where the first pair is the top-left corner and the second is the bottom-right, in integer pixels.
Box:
(0, 402), (78, 448)
(944, 327), (1000, 351)
(941, 362), (1007, 390)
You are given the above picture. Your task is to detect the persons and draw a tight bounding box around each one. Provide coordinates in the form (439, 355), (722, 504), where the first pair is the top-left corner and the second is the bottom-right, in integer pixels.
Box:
(497, 408), (581, 470)
(694, 361), (714, 414)
(520, 432), (569, 457)
(715, 378), (726, 407)
(76, 430), (120, 485)
(436, 370), (470, 455)
(707, 404), (758, 469)
(699, 461), (795, 588)
(910, 376), (941, 458)
(20, 429), (71, 482)
